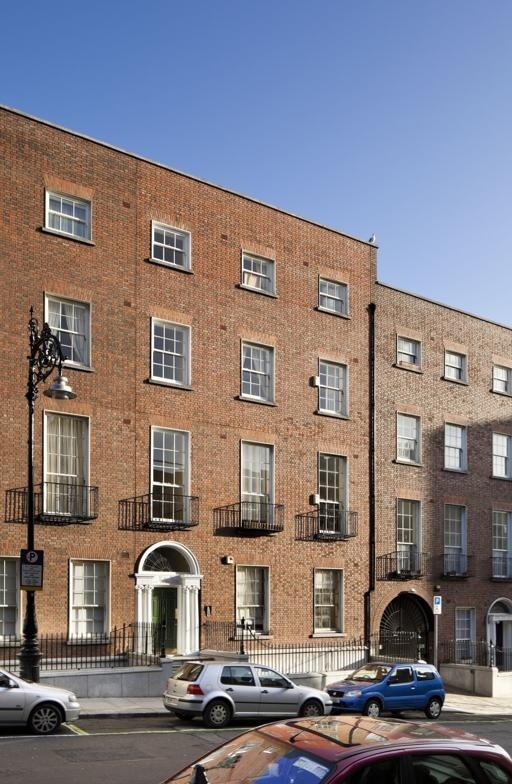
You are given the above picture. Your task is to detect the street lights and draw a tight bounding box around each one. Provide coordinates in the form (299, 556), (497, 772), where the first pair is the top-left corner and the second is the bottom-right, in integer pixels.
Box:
(16, 302), (79, 684)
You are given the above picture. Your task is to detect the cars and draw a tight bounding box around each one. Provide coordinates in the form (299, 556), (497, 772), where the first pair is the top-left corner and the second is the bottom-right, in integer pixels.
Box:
(160, 658), (334, 730)
(322, 659), (446, 721)
(0, 665), (81, 735)
(150, 712), (511, 783)
